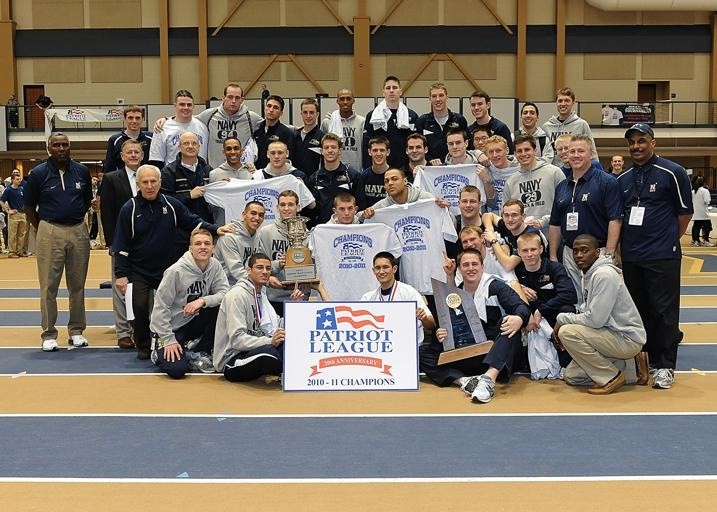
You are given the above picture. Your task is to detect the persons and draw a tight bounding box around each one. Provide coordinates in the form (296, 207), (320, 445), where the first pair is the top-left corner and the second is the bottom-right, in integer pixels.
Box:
(513, 232), (577, 373)
(113, 164), (238, 360)
(552, 233), (648, 395)
(0, 76), (717, 353)
(418, 248), (531, 403)
(213, 253), (285, 385)
(618, 122), (695, 388)
(150, 229), (231, 379)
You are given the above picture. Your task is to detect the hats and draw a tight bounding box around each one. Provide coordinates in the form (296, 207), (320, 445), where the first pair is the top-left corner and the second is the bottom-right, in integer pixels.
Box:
(622, 122), (656, 141)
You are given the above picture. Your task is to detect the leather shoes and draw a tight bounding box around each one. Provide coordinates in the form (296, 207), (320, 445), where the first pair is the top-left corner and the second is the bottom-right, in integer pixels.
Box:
(586, 371), (626, 395)
(634, 350), (649, 387)
(137, 347), (151, 360)
(117, 336), (135, 350)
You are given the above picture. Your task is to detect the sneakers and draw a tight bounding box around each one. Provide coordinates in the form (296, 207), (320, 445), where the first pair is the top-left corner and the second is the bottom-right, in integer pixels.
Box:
(42, 339), (59, 353)
(650, 367), (675, 389)
(19, 252), (28, 257)
(7, 252), (19, 258)
(690, 240), (703, 248)
(66, 333), (88, 349)
(459, 375), (477, 395)
(471, 373), (493, 403)
(260, 375), (280, 386)
(188, 351), (220, 376)
(702, 240), (715, 248)
(148, 331), (162, 366)
